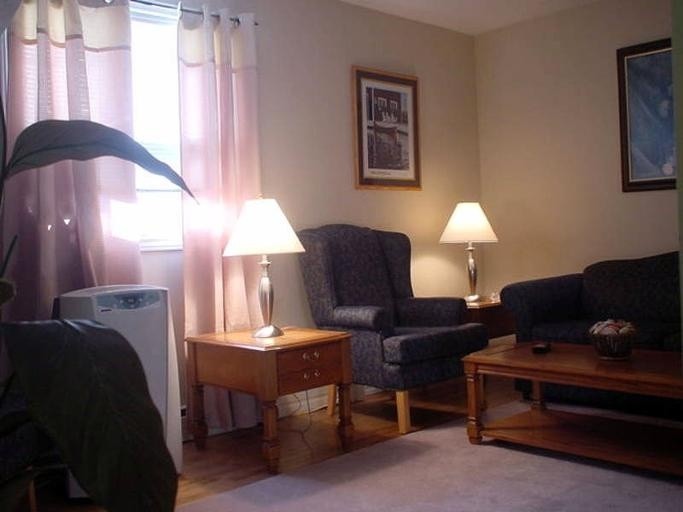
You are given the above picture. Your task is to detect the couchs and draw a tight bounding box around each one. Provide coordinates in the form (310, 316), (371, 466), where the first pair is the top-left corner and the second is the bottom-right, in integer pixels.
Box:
(499, 249), (683, 425)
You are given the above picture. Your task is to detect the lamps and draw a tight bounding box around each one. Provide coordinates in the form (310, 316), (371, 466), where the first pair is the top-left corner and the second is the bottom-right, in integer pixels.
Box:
(436, 199), (500, 301)
(222, 198), (306, 338)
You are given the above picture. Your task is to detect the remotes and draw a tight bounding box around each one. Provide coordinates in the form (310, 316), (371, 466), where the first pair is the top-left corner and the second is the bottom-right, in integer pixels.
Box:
(532, 342), (551, 352)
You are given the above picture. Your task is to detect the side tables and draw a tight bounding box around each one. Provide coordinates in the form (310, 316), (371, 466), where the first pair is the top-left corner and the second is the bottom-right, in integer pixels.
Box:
(184, 327), (354, 469)
(465, 301), (503, 323)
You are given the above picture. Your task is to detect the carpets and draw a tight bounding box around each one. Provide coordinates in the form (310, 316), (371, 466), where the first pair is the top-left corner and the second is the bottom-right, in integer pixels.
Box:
(177, 398), (683, 512)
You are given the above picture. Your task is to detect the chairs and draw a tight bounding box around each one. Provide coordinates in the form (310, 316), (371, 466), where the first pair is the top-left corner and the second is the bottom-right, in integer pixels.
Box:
(298, 225), (491, 436)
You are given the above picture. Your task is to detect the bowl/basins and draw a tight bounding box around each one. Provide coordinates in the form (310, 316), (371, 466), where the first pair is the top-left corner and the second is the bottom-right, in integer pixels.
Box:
(590, 333), (634, 360)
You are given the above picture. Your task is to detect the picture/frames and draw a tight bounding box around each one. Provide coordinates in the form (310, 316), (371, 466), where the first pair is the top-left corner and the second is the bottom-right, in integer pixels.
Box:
(351, 63), (423, 192)
(617, 36), (677, 193)
